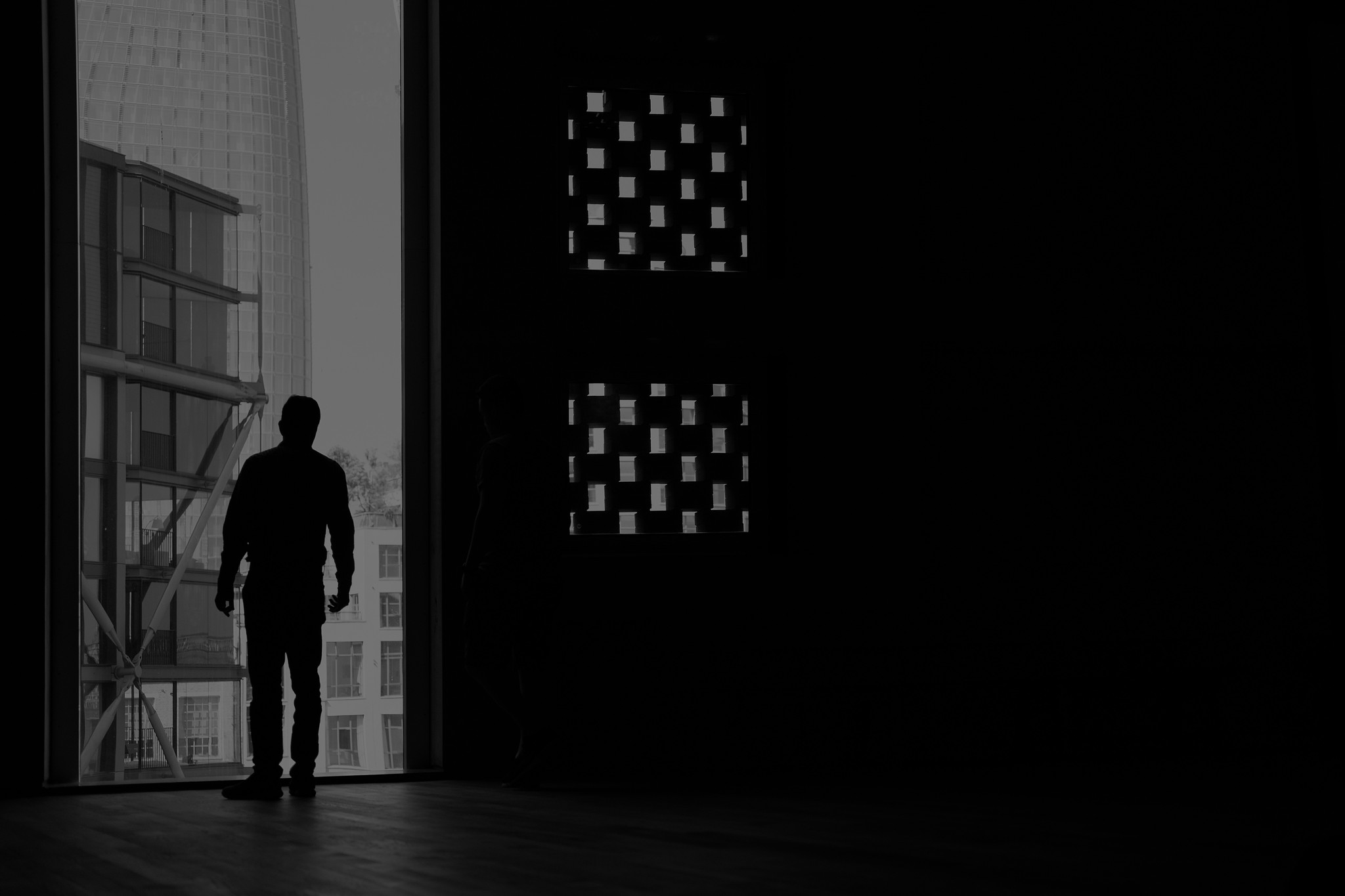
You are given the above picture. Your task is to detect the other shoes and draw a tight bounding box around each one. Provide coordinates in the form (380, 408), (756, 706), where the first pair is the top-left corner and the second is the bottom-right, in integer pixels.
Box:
(221, 775), (283, 800)
(289, 784), (315, 799)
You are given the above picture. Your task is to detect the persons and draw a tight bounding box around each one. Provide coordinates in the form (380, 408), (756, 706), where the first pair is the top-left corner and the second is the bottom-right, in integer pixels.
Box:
(212, 396), (355, 801)
